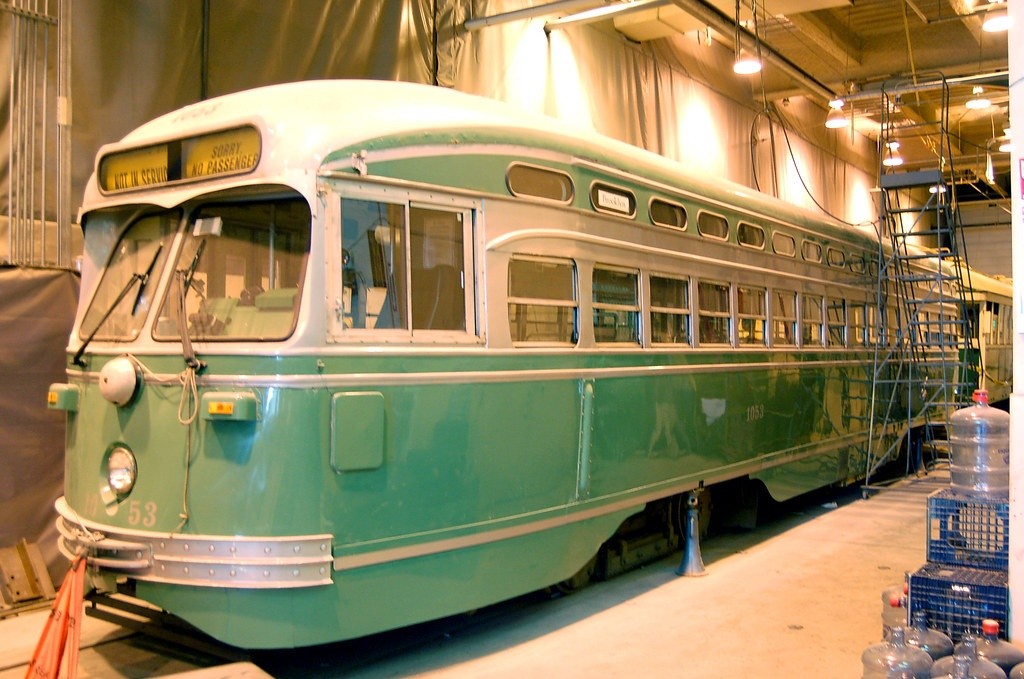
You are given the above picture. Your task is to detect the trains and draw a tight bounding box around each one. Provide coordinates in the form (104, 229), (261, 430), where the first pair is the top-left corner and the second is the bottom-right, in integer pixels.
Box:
(46, 77), (1009, 671)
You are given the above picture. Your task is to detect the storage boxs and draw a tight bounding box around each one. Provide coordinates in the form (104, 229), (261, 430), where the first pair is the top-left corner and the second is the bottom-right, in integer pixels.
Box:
(924, 488), (1008, 571)
(906, 564), (1009, 645)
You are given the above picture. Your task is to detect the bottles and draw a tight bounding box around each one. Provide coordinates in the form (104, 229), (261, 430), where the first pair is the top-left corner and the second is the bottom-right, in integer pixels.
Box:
(947, 390), (1009, 502)
(932, 645), (983, 679)
(881, 571), (912, 639)
(903, 609), (956, 659)
(932, 633), (1008, 679)
(1009, 662), (1024, 679)
(860, 626), (933, 679)
(953, 619), (1024, 679)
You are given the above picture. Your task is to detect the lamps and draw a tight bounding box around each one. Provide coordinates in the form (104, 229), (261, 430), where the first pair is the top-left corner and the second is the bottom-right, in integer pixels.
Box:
(928, 183), (947, 194)
(982, 3), (1008, 32)
(824, 98), (849, 129)
(965, 97), (990, 109)
(882, 141), (903, 166)
(732, 47), (761, 75)
(998, 140), (1010, 152)
